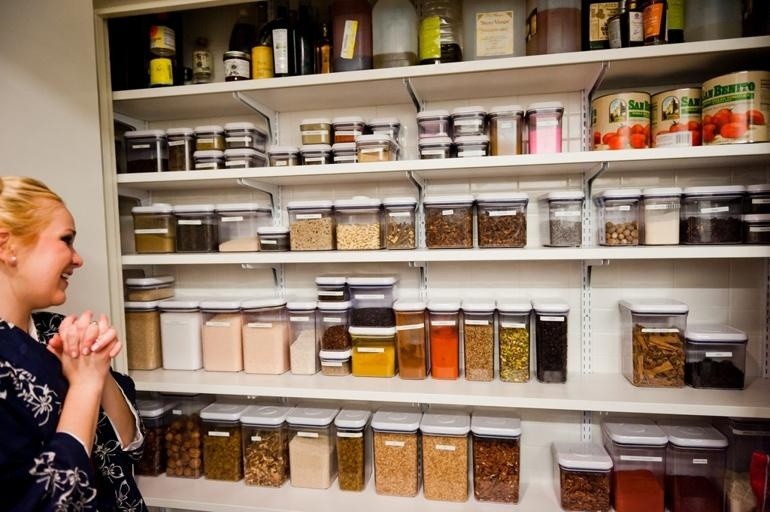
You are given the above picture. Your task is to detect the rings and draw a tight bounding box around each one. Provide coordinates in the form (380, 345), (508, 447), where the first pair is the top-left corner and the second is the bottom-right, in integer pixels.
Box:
(89, 320), (98, 325)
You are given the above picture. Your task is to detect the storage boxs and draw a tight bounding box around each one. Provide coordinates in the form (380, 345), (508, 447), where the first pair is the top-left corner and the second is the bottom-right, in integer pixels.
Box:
(420, 407), (471, 504)
(470, 410), (523, 504)
(551, 440), (613, 512)
(370, 407), (421, 497)
(128, 400), (375, 490)
(601, 412), (769, 512)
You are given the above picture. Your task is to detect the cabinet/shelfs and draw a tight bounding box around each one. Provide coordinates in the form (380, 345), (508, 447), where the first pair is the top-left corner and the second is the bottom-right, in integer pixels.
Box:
(94, 0), (770, 512)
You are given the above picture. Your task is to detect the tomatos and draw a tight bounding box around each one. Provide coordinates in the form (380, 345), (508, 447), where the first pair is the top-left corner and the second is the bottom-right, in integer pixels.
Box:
(592, 107), (764, 149)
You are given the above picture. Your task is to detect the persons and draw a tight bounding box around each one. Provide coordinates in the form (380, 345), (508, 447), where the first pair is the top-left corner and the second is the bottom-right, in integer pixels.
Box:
(0, 173), (153, 511)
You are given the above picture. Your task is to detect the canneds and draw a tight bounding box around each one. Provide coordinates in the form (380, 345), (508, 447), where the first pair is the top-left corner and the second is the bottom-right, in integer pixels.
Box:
(701, 72), (768, 144)
(650, 89), (702, 146)
(222, 51), (252, 81)
(591, 92), (651, 149)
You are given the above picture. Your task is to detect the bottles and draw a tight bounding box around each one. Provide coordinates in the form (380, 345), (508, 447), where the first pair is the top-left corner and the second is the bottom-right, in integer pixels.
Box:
(123, 178), (769, 248)
(606, 0), (685, 48)
(229, 0), (584, 81)
(125, 105), (573, 175)
(192, 35), (213, 84)
(126, 298), (754, 396)
(311, 271), (399, 303)
(126, 399), (770, 510)
(125, 276), (178, 304)
